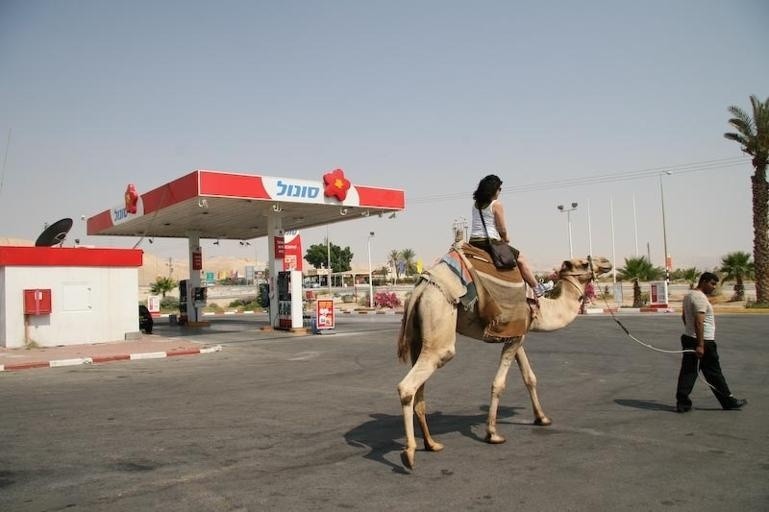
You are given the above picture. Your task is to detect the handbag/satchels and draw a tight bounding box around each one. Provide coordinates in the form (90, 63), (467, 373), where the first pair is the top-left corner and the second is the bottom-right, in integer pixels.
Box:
(489, 242), (516, 269)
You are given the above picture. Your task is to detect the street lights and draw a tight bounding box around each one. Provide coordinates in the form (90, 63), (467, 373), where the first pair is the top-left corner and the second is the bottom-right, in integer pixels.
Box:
(558, 203), (577, 260)
(368, 232), (374, 308)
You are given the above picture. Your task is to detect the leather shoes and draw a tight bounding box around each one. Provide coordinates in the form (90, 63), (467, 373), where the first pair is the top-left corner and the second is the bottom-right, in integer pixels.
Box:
(677, 399), (692, 413)
(722, 399), (747, 410)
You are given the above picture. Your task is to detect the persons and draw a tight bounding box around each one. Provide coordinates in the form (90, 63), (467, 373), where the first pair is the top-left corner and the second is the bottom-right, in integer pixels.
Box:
(674, 271), (748, 414)
(470, 173), (555, 298)
